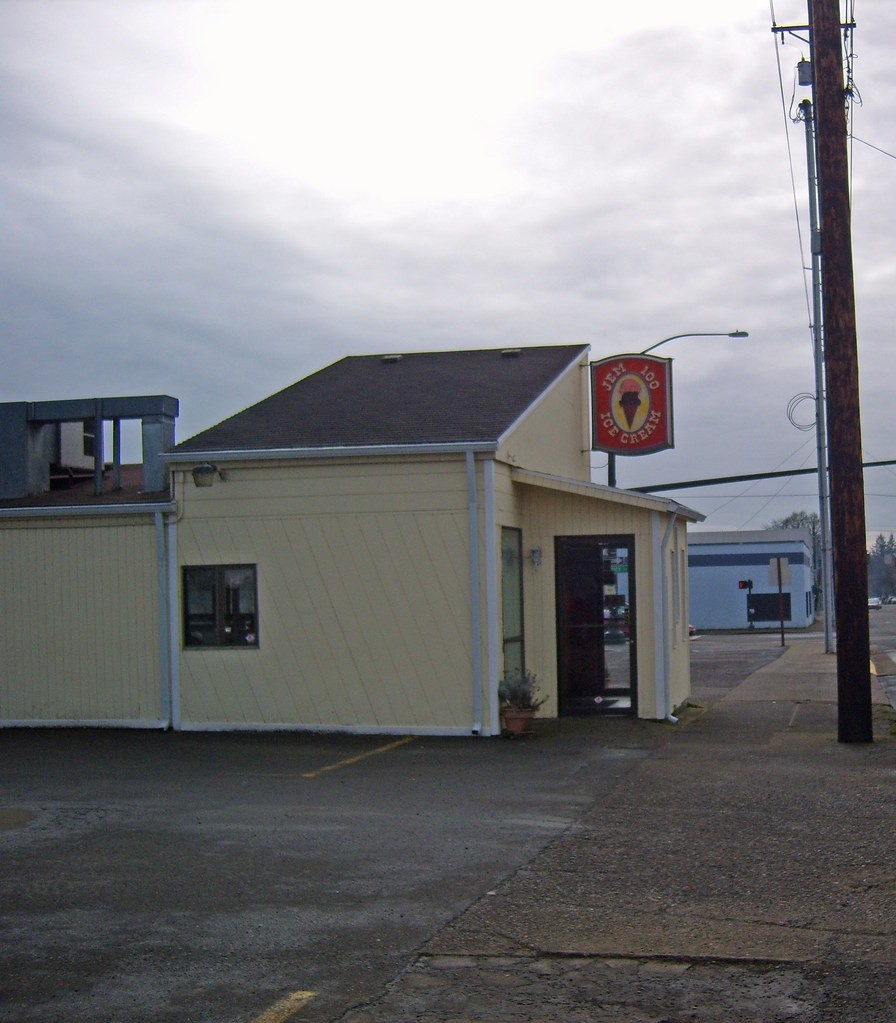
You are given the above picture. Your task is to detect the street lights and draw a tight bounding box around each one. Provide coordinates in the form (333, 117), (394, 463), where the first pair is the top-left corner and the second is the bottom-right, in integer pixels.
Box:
(608, 331), (749, 488)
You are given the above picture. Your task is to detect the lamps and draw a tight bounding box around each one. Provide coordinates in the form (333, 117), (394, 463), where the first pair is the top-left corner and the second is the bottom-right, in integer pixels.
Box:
(530, 543), (542, 567)
(217, 470), (232, 483)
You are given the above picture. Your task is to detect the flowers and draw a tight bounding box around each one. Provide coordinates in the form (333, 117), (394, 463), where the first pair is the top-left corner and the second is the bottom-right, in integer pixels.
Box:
(498, 666), (549, 709)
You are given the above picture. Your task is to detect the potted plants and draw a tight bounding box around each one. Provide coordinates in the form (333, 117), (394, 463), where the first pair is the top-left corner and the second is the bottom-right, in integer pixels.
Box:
(192, 466), (213, 488)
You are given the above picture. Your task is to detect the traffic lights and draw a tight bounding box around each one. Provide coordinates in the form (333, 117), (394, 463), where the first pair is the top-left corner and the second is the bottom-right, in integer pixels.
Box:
(738, 581), (747, 589)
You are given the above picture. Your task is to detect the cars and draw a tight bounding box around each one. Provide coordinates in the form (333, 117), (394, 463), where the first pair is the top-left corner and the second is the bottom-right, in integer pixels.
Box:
(886, 595), (896, 604)
(868, 598), (882, 610)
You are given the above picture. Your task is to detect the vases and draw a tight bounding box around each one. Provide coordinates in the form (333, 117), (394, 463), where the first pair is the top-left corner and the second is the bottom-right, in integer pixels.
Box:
(503, 707), (537, 733)
